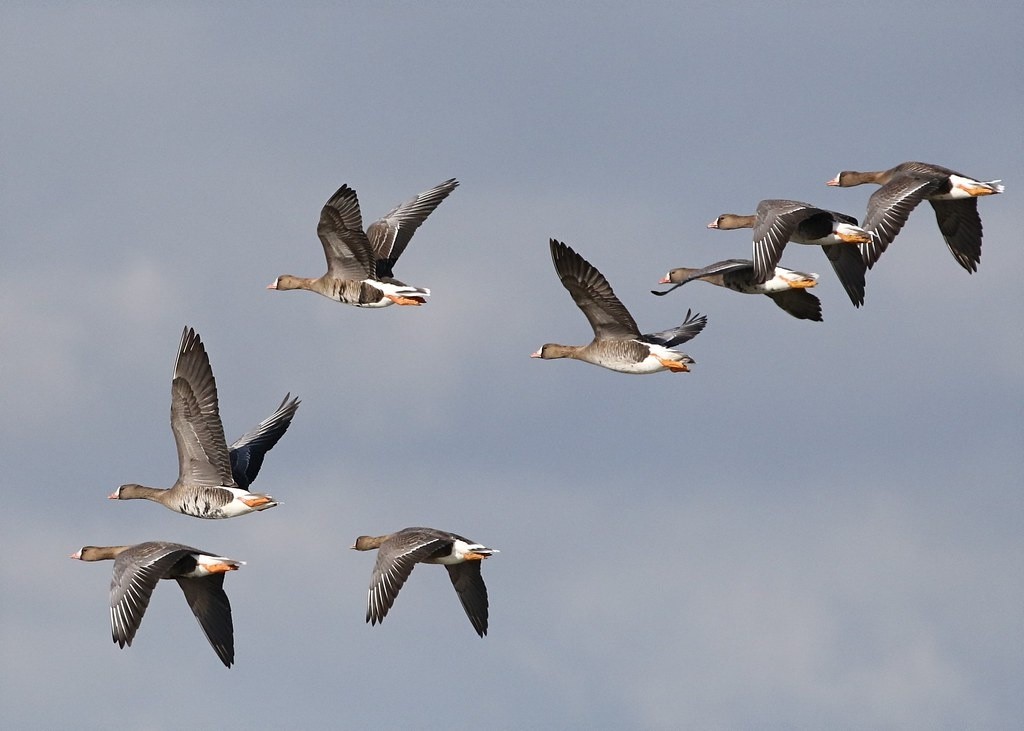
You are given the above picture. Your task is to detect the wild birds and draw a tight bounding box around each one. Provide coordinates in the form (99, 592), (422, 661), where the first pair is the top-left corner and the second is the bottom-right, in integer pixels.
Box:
(650, 259), (823, 325)
(70, 541), (248, 671)
(351, 528), (498, 641)
(109, 324), (302, 521)
(265, 178), (461, 309)
(827, 161), (1005, 274)
(530, 238), (709, 376)
(707, 199), (868, 310)
